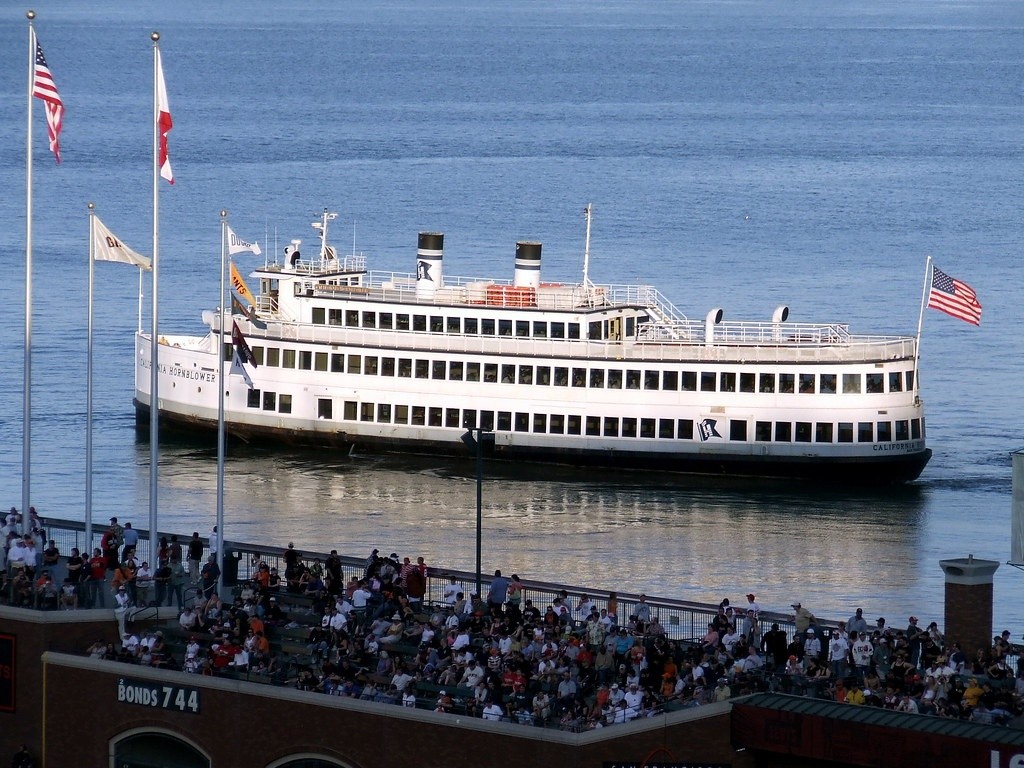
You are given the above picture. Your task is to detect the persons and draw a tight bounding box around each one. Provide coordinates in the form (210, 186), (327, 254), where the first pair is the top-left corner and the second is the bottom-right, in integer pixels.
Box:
(0, 507), (1024, 733)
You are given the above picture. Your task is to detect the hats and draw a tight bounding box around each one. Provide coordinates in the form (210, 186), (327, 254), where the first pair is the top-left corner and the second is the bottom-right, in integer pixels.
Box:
(790, 600), (802, 606)
(119, 586), (127, 591)
(935, 658), (944, 664)
(880, 638), (887, 642)
(565, 626), (571, 634)
(789, 655), (796, 660)
(859, 632), (866, 637)
(154, 631), (162, 636)
(909, 617), (918, 621)
(807, 629), (814, 633)
(590, 606), (596, 610)
(876, 618), (885, 623)
(391, 614), (401, 620)
(746, 594), (755, 600)
(612, 684), (618, 688)
(968, 678), (978, 684)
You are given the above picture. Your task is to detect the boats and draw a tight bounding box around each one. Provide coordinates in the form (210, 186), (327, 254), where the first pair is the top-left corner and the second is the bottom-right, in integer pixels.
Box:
(133, 203), (932, 490)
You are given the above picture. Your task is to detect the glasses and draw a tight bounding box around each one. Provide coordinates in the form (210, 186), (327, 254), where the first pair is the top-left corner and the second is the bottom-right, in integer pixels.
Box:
(582, 599), (584, 600)
(440, 694), (445, 696)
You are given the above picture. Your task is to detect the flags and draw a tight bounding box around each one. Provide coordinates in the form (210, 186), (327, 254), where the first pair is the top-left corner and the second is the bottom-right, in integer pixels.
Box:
(32, 29), (65, 163)
(924, 264), (982, 327)
(227, 225), (267, 390)
(92, 215), (153, 270)
(155, 47), (174, 185)
